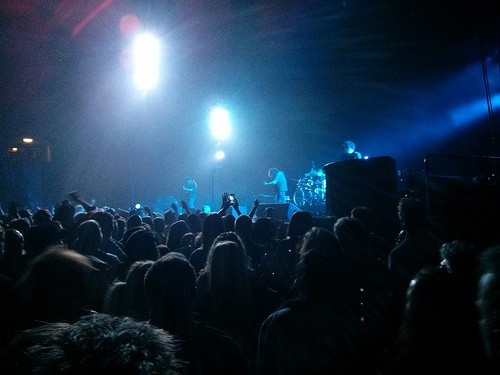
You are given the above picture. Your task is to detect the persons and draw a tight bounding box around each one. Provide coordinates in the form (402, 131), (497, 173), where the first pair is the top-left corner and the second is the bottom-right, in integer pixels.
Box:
(183, 176), (198, 208)
(264, 168), (288, 202)
(0, 190), (500, 375)
(342, 140), (361, 160)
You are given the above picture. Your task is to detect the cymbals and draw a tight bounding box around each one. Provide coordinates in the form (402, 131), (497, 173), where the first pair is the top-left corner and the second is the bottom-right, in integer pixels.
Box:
(305, 171), (321, 175)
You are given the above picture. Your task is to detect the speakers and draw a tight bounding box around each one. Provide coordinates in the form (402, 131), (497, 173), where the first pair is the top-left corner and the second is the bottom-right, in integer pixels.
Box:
(256, 203), (300, 222)
(321, 155), (397, 216)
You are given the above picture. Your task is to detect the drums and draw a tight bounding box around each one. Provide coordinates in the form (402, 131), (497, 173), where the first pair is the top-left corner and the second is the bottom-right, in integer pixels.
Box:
(298, 179), (312, 189)
(293, 190), (312, 206)
(306, 176), (321, 185)
